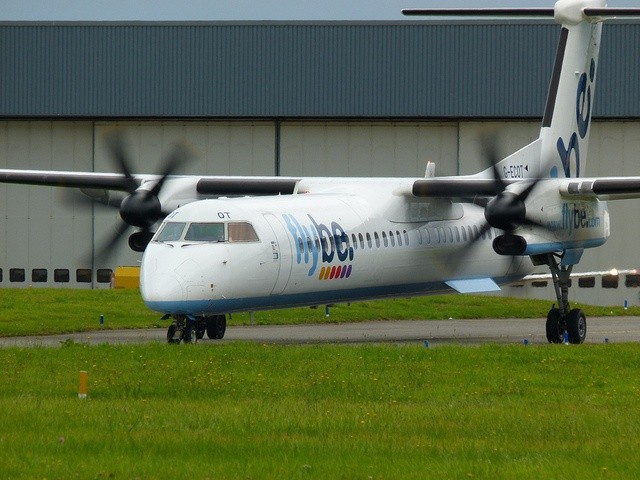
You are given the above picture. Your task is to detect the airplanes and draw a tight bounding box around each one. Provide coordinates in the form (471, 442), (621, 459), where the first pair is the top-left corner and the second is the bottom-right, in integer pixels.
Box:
(0, 0), (640, 345)
